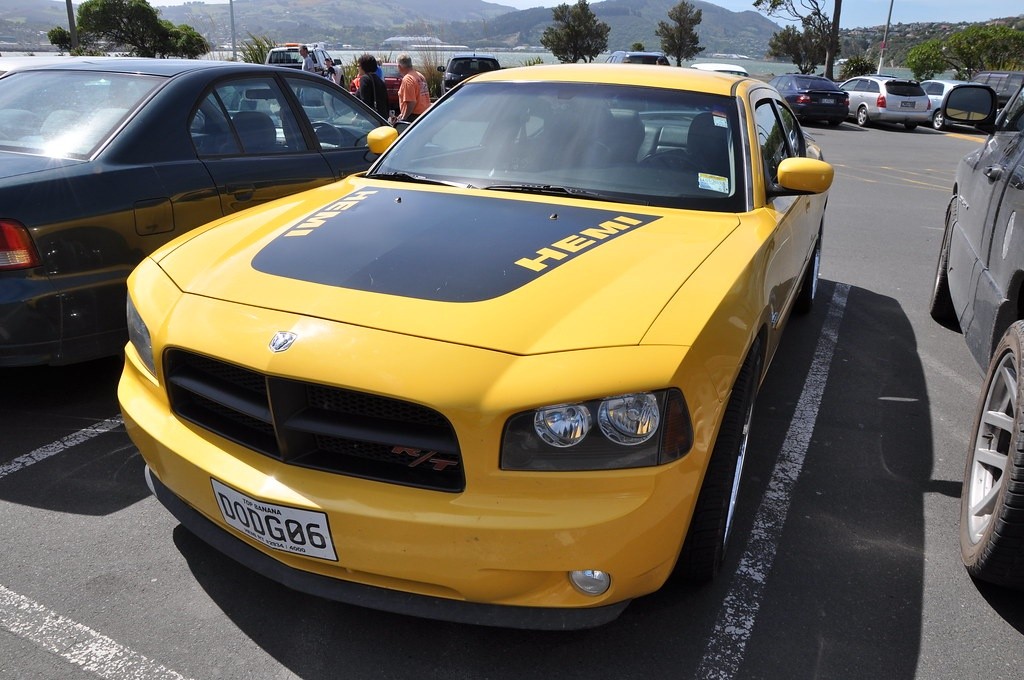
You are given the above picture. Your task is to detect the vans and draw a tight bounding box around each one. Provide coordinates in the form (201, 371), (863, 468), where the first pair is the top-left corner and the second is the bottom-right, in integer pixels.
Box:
(971, 70), (1024, 109)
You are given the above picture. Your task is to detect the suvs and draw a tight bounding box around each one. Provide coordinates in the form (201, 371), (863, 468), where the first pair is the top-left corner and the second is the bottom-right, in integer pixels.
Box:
(604, 50), (671, 67)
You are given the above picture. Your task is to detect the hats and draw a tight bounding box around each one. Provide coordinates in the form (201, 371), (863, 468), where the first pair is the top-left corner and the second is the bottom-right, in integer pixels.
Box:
(325, 57), (330, 61)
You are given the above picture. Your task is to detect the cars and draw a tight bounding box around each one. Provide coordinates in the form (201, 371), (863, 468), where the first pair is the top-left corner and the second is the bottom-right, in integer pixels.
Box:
(349, 63), (404, 104)
(436, 54), (507, 98)
(838, 75), (932, 131)
(691, 63), (749, 78)
(116, 63), (833, 630)
(921, 78), (991, 130)
(930, 77), (1024, 594)
(1, 57), (445, 369)
(767, 73), (850, 126)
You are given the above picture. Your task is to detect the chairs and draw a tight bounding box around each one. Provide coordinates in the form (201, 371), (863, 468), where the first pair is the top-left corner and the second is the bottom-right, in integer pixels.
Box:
(657, 113), (729, 197)
(217, 110), (287, 158)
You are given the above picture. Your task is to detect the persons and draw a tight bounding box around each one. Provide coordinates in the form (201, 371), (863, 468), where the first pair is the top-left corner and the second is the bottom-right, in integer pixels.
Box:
(388, 53), (430, 123)
(298, 45), (316, 73)
(324, 54), (389, 122)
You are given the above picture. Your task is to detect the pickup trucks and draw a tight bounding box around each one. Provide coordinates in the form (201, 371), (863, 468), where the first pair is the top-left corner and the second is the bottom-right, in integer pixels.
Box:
(264, 42), (345, 88)
